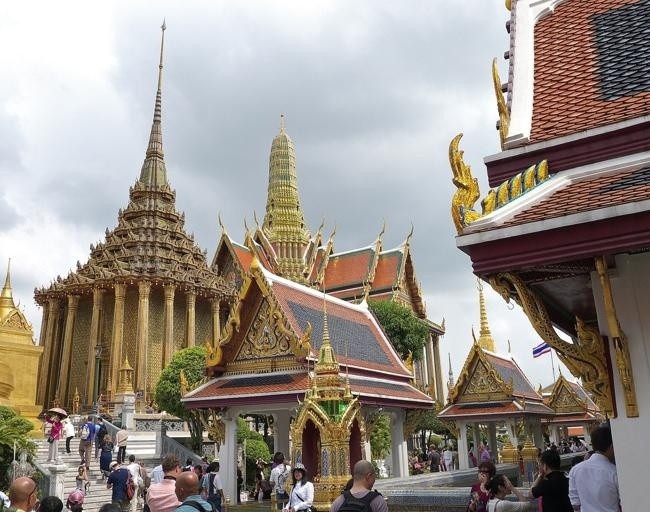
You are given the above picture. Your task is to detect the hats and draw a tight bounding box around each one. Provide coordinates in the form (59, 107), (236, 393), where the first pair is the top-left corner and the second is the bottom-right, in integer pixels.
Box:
(69, 491), (84, 504)
(292, 463), (307, 471)
(109, 461), (118, 470)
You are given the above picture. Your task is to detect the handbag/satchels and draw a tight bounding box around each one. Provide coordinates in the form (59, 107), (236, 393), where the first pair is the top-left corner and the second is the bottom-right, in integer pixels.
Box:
(47, 436), (53, 443)
(311, 506), (317, 512)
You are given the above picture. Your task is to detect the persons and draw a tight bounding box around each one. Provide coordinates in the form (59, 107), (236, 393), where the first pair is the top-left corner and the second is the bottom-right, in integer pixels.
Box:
(2, 405), (588, 511)
(568, 426), (622, 511)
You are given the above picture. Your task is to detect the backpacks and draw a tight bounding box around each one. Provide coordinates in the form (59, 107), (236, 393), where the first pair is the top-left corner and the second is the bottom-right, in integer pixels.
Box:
(336, 491), (379, 512)
(80, 424), (90, 439)
(127, 471), (135, 499)
